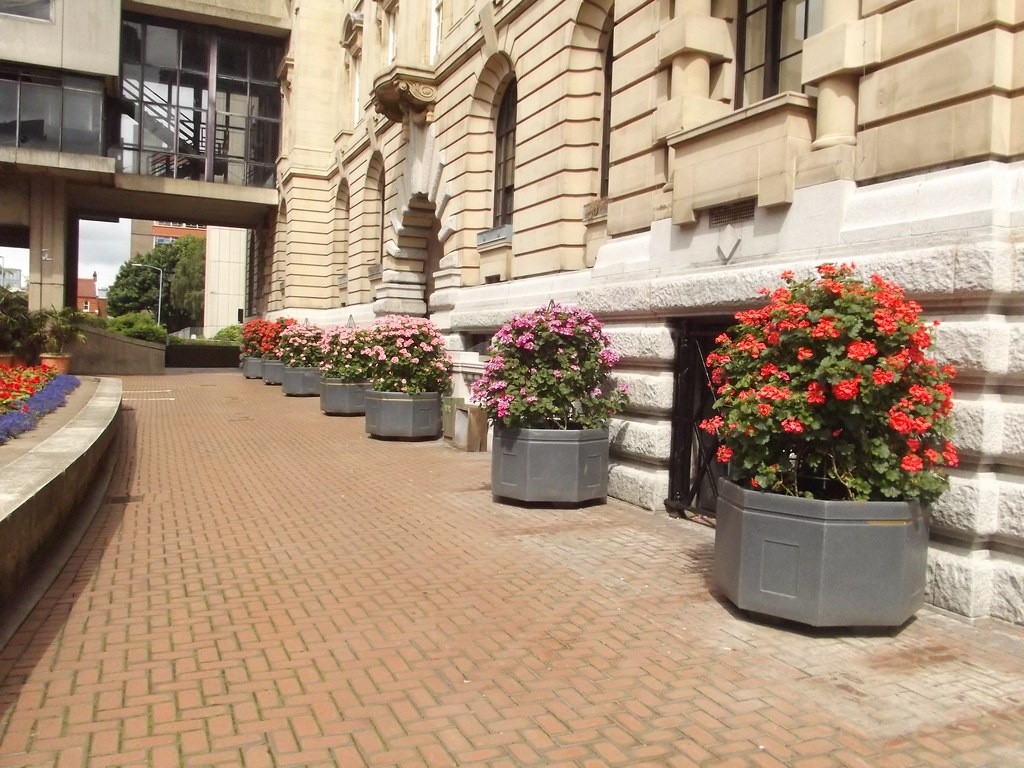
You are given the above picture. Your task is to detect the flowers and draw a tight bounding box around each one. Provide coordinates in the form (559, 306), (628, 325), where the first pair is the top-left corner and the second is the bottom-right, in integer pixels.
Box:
(698, 260), (960, 504)
(239, 314), (455, 398)
(470, 300), (630, 430)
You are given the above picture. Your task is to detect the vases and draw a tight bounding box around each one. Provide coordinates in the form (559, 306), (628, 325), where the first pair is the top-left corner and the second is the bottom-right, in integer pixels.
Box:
(282, 367), (322, 396)
(320, 377), (374, 416)
(242, 357), (263, 381)
(712, 476), (933, 630)
(364, 387), (441, 439)
(491, 420), (609, 504)
(261, 360), (283, 385)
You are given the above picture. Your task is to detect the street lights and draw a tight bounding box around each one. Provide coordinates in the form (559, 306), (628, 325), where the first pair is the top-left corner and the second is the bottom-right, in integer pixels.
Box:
(131, 263), (163, 326)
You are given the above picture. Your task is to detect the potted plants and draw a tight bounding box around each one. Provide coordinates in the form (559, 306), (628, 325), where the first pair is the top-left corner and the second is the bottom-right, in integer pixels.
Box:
(27, 303), (87, 375)
